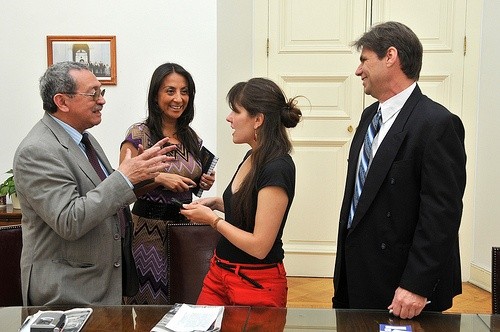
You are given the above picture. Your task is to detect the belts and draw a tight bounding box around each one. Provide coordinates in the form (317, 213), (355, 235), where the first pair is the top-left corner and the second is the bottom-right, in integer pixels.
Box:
(131, 198), (192, 222)
(214, 257), (278, 288)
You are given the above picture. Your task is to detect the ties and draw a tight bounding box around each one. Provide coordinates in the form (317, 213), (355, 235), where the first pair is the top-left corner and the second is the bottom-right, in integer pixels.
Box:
(346, 108), (383, 229)
(80, 134), (126, 238)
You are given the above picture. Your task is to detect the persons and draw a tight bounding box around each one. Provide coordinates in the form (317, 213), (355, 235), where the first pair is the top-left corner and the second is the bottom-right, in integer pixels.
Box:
(179, 78), (311, 305)
(117, 63), (215, 305)
(13, 61), (176, 304)
(331, 23), (467, 319)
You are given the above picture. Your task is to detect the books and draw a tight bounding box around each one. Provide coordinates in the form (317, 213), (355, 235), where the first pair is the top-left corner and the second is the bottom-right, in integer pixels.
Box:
(191, 148), (219, 198)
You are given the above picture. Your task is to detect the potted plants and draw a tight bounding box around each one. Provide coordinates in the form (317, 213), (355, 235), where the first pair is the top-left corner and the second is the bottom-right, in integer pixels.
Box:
(0, 169), (22, 209)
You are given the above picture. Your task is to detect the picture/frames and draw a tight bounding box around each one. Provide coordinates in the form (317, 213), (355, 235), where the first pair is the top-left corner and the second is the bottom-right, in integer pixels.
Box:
(47, 35), (116, 85)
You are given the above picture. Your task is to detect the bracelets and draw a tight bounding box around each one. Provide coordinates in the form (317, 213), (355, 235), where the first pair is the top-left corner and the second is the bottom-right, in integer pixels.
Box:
(212, 217), (225, 232)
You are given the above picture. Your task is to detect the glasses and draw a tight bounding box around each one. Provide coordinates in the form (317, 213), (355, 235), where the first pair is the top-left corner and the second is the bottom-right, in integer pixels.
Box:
(60, 89), (107, 101)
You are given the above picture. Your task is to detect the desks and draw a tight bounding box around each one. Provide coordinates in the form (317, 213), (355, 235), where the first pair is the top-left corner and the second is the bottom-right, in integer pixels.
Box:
(0, 306), (500, 332)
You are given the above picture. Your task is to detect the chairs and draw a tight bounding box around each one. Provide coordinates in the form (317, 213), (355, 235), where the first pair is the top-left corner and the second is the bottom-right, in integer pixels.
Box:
(167, 223), (219, 305)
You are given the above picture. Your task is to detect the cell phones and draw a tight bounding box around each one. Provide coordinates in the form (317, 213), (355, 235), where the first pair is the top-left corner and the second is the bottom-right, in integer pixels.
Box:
(31, 312), (66, 332)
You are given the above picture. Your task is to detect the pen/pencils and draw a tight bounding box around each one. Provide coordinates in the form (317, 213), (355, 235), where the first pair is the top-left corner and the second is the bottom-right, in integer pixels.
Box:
(171, 198), (185, 209)
(163, 185), (196, 191)
(389, 301), (432, 313)
